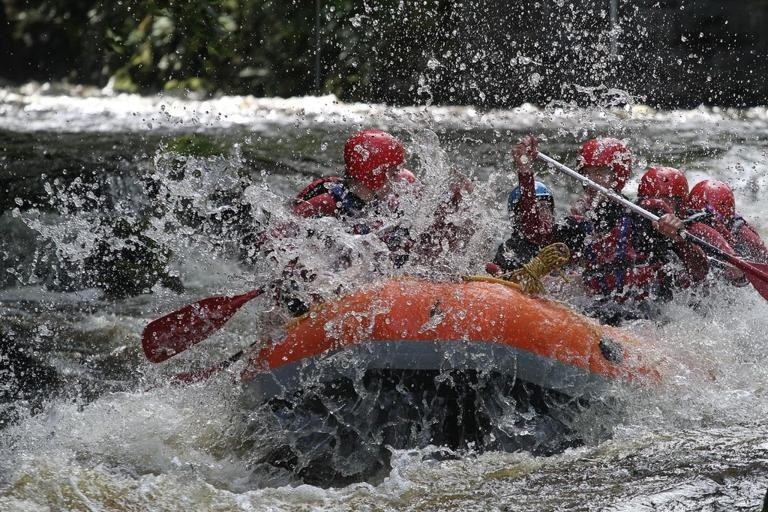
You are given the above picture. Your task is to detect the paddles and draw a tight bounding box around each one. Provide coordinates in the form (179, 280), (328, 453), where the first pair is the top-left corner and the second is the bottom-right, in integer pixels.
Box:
(145, 342), (257, 394)
(141, 278), (285, 364)
(529, 147), (768, 303)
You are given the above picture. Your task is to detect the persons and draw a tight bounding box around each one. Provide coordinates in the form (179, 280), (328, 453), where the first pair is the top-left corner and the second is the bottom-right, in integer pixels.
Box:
(255, 129), (768, 325)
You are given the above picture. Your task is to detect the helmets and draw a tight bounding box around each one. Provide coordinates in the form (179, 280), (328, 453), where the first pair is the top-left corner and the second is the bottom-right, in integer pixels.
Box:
(343, 132), (403, 191)
(637, 166), (688, 208)
(688, 180), (735, 223)
(577, 137), (631, 191)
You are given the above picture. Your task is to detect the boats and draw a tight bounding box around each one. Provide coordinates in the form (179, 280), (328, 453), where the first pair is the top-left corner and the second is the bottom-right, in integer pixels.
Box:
(240, 281), (663, 487)
